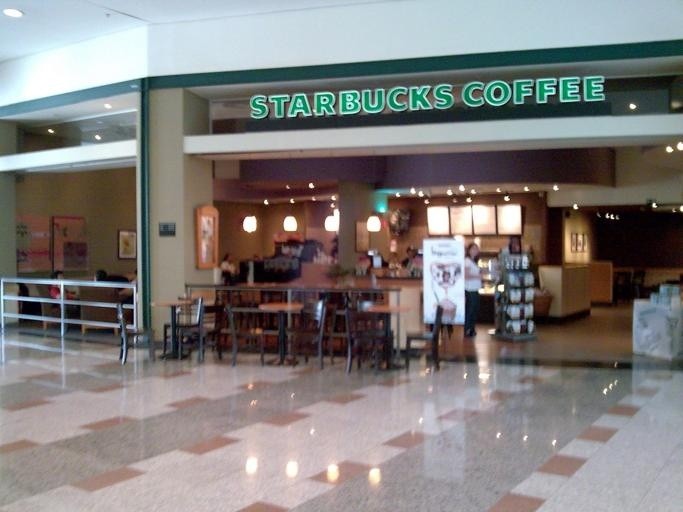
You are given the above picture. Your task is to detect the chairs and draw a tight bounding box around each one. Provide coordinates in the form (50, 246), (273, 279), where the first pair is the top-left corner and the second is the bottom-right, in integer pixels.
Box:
(609, 267), (650, 307)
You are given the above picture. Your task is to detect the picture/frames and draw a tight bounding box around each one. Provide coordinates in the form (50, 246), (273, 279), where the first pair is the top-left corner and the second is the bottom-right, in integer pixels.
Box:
(195, 202), (220, 270)
(569, 231), (590, 253)
(354, 220), (372, 254)
(116, 227), (137, 261)
(507, 234), (523, 255)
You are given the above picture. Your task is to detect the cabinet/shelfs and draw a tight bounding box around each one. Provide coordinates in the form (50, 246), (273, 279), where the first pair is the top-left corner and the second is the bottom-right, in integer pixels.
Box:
(538, 261), (594, 327)
(630, 295), (683, 366)
(473, 248), (501, 326)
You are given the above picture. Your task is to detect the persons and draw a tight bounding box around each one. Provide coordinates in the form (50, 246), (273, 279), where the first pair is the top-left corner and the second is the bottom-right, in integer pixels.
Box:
(219, 253), (236, 275)
(47, 270), (79, 309)
(366, 245), (423, 280)
(464, 243), (485, 337)
(117, 268), (137, 298)
(439, 272), (452, 285)
